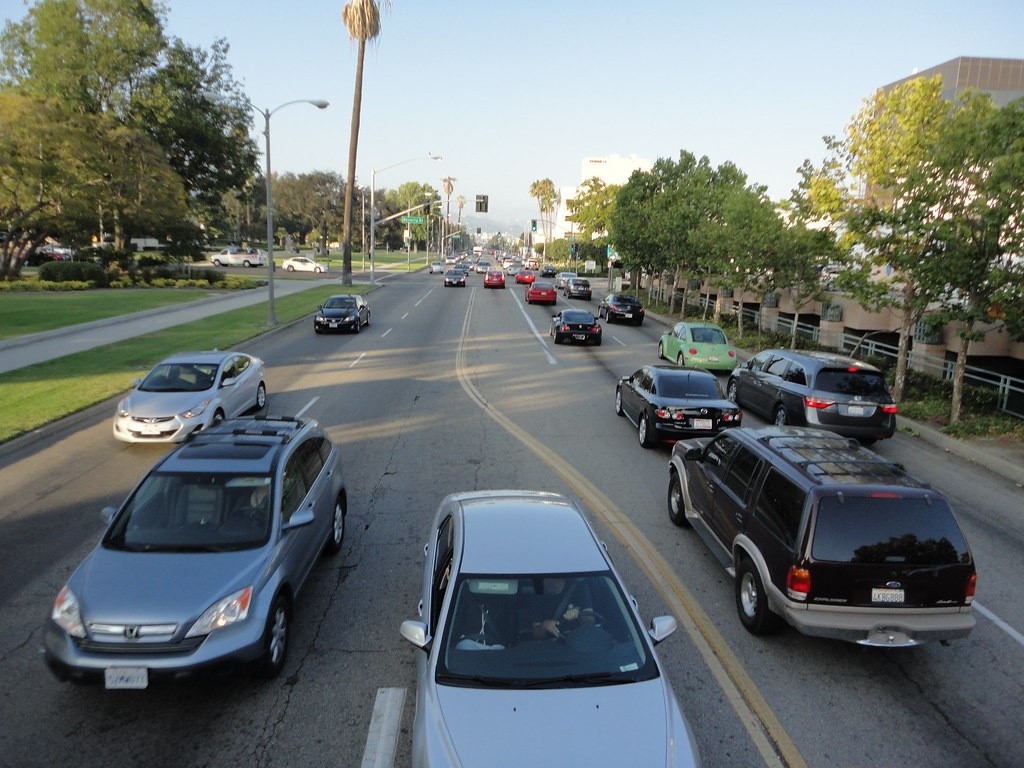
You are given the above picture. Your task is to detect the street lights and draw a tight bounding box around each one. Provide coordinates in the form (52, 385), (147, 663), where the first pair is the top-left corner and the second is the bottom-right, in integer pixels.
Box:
(202, 91), (329, 325)
(426, 206), (443, 265)
(407, 192), (432, 269)
(438, 213), (453, 254)
(370, 152), (442, 285)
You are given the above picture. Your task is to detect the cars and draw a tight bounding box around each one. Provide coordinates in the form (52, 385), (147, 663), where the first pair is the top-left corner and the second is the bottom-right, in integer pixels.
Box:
(598, 294), (644, 325)
(399, 487), (704, 768)
(524, 282), (557, 306)
(281, 257), (328, 274)
(563, 278), (592, 300)
(429, 246), (556, 289)
(615, 365), (744, 449)
(111, 347), (269, 443)
(555, 272), (577, 289)
(20, 248), (69, 267)
(657, 321), (737, 374)
(313, 295), (370, 335)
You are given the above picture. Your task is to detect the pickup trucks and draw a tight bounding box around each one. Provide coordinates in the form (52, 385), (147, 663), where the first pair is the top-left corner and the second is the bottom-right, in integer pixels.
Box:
(211, 247), (266, 268)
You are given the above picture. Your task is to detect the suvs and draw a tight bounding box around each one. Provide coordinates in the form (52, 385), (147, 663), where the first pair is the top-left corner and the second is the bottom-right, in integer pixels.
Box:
(667, 422), (977, 649)
(42, 414), (348, 686)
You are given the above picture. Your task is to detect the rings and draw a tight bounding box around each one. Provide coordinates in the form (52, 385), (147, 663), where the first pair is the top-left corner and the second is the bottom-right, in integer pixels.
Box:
(572, 612), (576, 615)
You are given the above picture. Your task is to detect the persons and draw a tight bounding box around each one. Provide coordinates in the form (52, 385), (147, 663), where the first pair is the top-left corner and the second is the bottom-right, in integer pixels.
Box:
(529, 577), (599, 638)
(231, 484), (273, 527)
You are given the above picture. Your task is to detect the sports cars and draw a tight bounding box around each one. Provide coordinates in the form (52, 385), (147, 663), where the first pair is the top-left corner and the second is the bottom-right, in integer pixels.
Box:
(549, 308), (603, 346)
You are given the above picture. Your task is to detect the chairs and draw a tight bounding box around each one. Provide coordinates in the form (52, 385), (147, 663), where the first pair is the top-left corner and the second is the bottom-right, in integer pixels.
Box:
(331, 299), (342, 307)
(176, 366), (199, 389)
(173, 485), (222, 532)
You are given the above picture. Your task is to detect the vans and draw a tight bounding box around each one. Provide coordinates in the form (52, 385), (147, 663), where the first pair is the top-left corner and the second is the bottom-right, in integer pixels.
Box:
(726, 347), (896, 446)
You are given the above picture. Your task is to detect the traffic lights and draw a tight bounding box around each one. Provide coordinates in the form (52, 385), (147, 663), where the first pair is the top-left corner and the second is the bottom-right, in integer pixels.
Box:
(575, 243), (578, 252)
(531, 219), (537, 231)
(571, 243), (575, 253)
